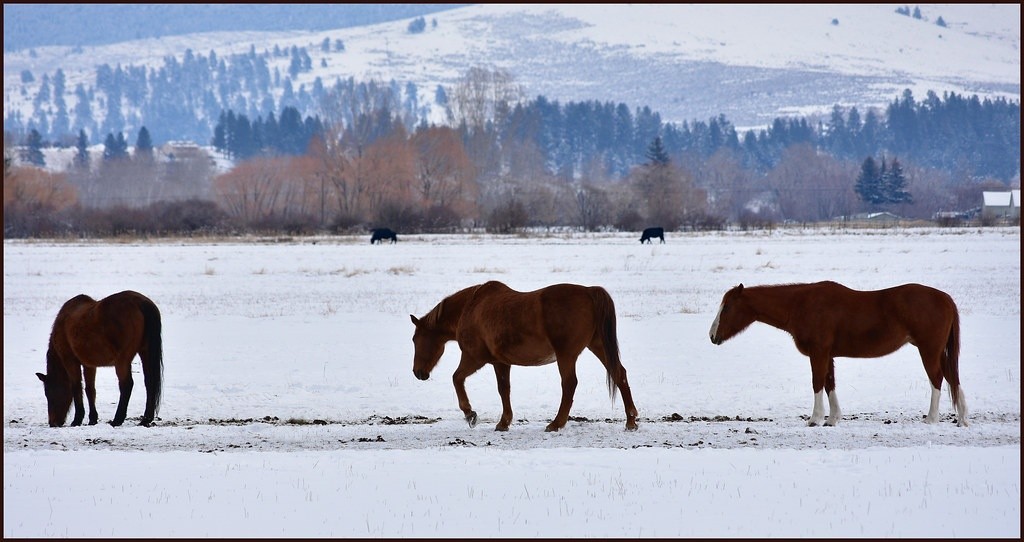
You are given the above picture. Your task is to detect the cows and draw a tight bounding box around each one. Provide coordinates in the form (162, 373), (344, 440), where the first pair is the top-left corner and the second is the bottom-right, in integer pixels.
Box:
(639, 227), (665, 244)
(371, 227), (397, 244)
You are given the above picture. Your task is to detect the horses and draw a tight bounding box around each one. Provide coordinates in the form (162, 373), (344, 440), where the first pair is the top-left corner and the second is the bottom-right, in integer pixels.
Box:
(36, 290), (162, 427)
(410, 281), (638, 431)
(709, 280), (968, 426)
